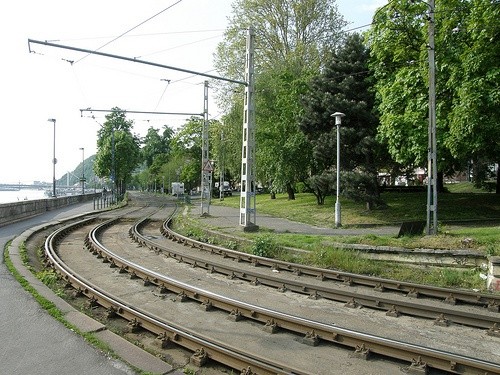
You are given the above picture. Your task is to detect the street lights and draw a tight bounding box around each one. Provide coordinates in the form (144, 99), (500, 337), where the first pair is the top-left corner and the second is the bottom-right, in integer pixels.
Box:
(331, 112), (346, 228)
(79, 147), (84, 194)
(48, 118), (56, 198)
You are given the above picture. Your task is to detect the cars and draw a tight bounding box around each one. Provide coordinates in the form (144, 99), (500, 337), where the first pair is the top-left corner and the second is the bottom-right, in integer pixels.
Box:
(219, 186), (233, 197)
